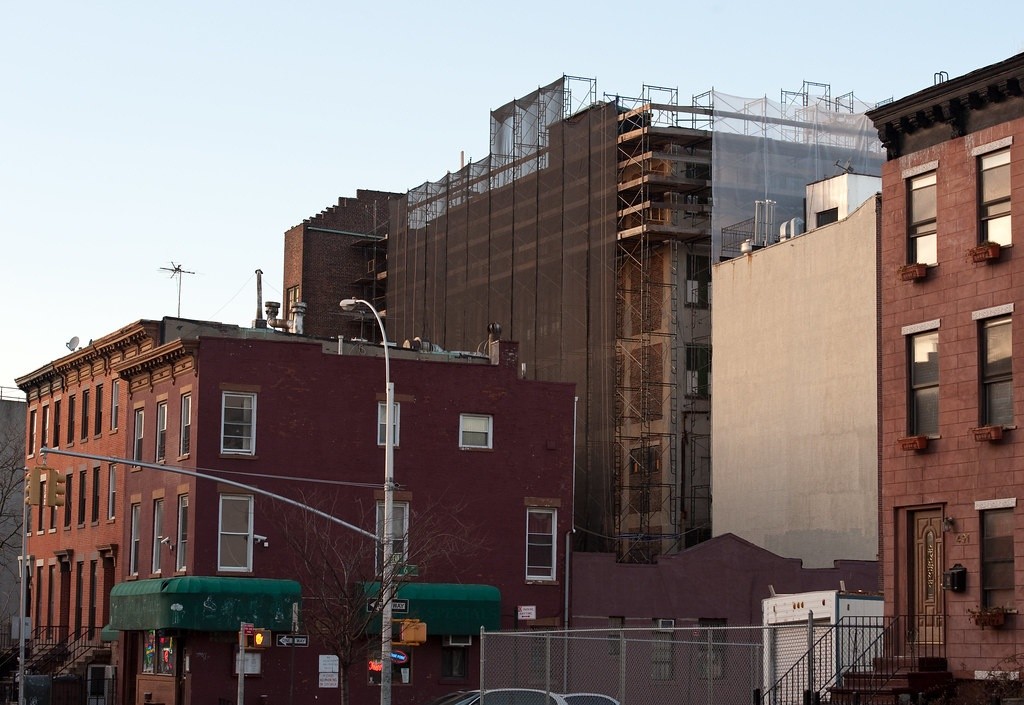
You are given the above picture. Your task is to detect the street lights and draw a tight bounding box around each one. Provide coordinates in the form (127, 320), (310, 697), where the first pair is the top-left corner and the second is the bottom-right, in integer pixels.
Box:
(341, 298), (396, 705)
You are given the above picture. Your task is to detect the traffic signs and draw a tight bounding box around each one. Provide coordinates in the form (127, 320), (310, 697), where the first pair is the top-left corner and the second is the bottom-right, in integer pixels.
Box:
(276, 634), (310, 648)
(366, 597), (410, 615)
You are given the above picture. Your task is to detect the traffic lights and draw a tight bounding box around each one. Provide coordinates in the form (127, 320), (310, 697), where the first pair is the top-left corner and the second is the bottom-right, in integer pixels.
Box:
(46, 469), (66, 508)
(254, 631), (270, 647)
(24, 469), (40, 506)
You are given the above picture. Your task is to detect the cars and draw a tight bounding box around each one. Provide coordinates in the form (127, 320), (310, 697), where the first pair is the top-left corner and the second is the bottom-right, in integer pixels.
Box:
(436, 690), (481, 705)
(431, 691), (465, 705)
(456, 688), (568, 705)
(558, 692), (621, 705)
